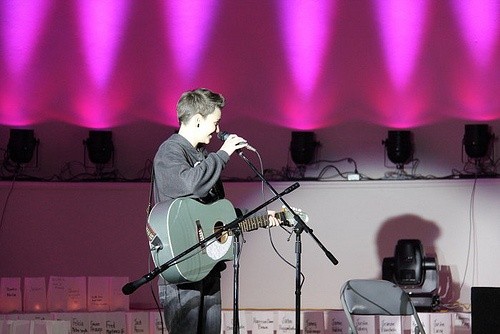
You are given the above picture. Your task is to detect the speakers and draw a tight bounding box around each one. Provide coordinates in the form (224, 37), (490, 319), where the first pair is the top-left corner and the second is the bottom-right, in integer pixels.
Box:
(292, 132), (316, 164)
(464, 123), (489, 157)
(86, 131), (112, 163)
(386, 130), (414, 164)
(6, 128), (35, 162)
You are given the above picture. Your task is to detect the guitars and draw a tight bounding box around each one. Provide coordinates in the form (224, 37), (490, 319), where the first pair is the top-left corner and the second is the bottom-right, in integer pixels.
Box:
(146, 197), (309, 284)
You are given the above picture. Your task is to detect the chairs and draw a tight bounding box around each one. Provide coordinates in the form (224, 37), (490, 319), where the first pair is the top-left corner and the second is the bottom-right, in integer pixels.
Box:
(341, 278), (427, 334)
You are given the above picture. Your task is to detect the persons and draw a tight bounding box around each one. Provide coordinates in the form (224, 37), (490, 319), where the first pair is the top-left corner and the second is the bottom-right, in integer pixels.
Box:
(150, 88), (283, 334)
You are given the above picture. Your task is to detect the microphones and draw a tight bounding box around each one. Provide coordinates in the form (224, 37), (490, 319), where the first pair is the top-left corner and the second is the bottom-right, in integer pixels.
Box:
(122, 268), (161, 295)
(217, 131), (256, 152)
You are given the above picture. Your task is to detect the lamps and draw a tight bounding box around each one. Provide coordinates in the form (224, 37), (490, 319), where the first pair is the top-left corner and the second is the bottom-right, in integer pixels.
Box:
(82, 130), (115, 180)
(289, 132), (318, 179)
(461, 124), (498, 176)
(382, 131), (417, 180)
(381, 238), (440, 312)
(2, 128), (40, 181)
